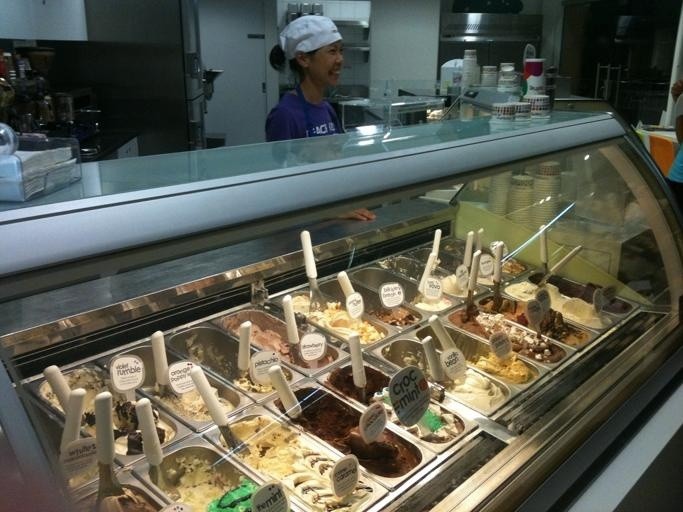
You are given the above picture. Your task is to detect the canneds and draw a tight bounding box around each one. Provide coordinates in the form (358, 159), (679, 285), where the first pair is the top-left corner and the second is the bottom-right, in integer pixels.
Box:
(54, 93), (73, 122)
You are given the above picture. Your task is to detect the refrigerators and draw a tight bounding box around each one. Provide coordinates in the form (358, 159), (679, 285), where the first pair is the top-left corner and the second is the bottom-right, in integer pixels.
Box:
(81, 0), (206, 153)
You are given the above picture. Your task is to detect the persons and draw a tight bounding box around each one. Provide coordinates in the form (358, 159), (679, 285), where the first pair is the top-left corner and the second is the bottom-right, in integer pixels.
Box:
(262, 14), (378, 241)
(665, 91), (683, 212)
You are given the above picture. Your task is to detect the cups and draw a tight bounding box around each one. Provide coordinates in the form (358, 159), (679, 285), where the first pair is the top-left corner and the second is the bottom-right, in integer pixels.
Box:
(486, 161), (563, 231)
(2, 52), (16, 80)
(489, 93), (552, 123)
(461, 49), (548, 97)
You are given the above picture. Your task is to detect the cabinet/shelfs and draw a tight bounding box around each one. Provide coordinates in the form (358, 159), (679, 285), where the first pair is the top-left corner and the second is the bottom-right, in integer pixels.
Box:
(0, 106), (683, 512)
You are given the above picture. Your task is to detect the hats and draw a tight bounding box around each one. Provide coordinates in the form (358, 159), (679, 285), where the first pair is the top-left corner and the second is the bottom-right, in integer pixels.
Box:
(278, 14), (343, 60)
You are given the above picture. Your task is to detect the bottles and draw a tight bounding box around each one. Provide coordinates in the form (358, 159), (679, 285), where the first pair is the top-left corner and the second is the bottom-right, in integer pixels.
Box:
(17, 60), (26, 80)
